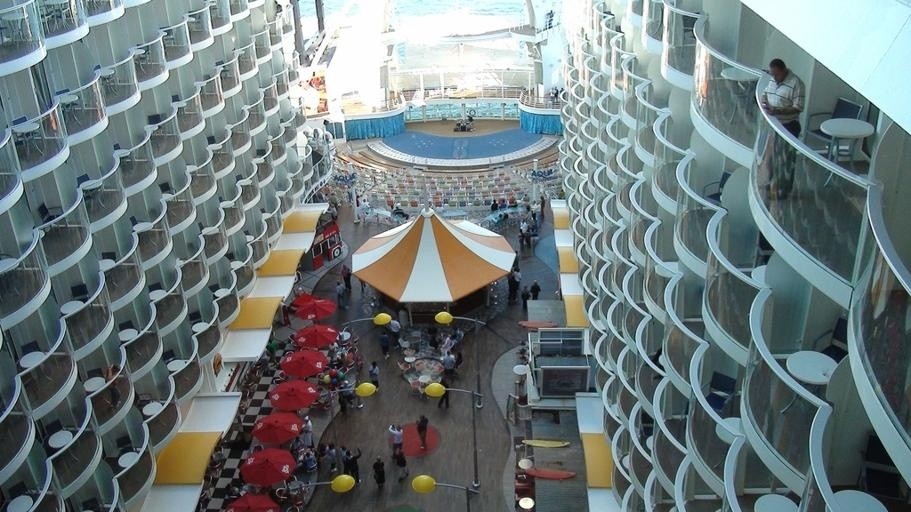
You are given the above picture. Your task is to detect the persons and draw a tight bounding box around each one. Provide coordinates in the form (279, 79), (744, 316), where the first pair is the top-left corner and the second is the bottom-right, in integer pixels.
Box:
(373, 456), (386, 489)
(327, 442), (362, 485)
(442, 350), (463, 382)
(305, 452), (316, 464)
(548, 87), (565, 103)
(438, 375), (450, 408)
(345, 359), (363, 409)
(467, 116), (474, 126)
(324, 187), (545, 308)
(380, 331), (390, 359)
(761, 58), (804, 200)
(396, 451), (409, 480)
(303, 416), (315, 449)
(429, 332), (441, 349)
(417, 415), (429, 449)
(389, 424), (404, 458)
(390, 318), (401, 350)
(450, 327), (458, 340)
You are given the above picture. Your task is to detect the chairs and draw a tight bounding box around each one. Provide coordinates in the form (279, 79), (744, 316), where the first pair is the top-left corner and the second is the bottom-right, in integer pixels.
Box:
(358, 161), (535, 219)
(392, 323), (467, 403)
(1, 3), (285, 512)
(623, 1), (910, 512)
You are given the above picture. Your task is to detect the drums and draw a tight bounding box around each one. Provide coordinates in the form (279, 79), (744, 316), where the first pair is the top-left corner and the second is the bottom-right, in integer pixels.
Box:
(468, 122), (471, 130)
(457, 120), (461, 127)
(461, 123), (467, 131)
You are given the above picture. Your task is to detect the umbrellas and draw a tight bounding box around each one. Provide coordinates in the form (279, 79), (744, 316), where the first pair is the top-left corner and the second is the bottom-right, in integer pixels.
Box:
(229, 295), (339, 512)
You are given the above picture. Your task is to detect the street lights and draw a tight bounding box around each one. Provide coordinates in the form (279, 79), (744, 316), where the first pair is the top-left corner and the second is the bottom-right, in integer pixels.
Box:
(320, 382), (377, 475)
(435, 311), (486, 410)
(281, 474), (356, 511)
(342, 312), (392, 410)
(424, 382), (483, 489)
(411, 475), (479, 512)
